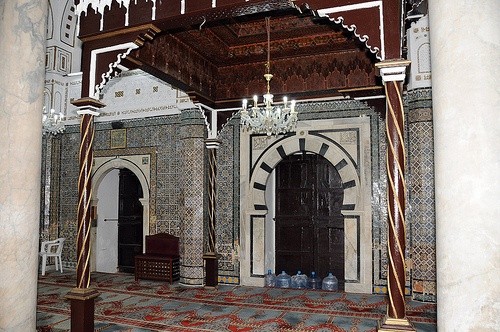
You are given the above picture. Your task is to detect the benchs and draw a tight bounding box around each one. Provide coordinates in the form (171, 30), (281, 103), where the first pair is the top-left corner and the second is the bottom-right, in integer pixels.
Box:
(133, 233), (181, 285)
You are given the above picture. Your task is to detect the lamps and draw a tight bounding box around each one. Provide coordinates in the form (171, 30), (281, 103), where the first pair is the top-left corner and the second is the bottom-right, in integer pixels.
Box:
(42, 91), (66, 137)
(239, 16), (299, 138)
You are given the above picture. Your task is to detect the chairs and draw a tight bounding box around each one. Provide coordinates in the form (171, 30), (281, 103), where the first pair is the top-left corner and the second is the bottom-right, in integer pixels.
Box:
(39, 237), (67, 276)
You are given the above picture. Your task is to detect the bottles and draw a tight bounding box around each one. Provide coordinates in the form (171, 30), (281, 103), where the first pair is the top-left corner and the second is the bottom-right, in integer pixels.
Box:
(308, 271), (321, 290)
(322, 273), (338, 291)
(290, 270), (307, 289)
(276, 271), (291, 289)
(265, 270), (276, 287)
